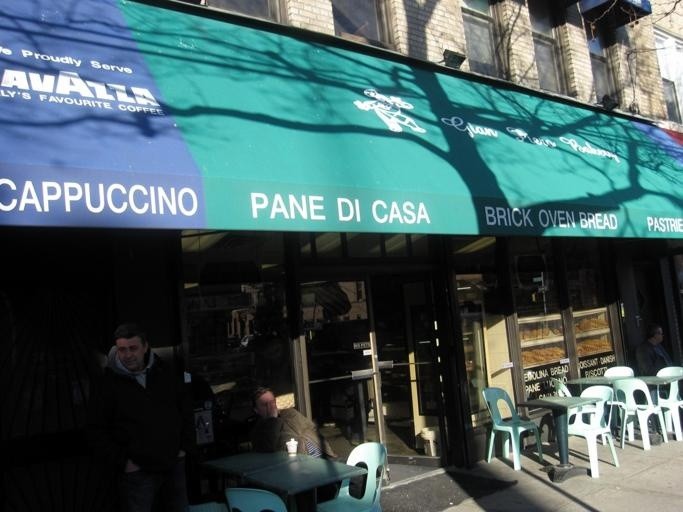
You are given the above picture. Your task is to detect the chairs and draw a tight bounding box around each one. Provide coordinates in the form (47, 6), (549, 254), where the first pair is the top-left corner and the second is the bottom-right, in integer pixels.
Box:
(478, 366), (682, 478)
(316, 441), (388, 512)
(224, 488), (288, 512)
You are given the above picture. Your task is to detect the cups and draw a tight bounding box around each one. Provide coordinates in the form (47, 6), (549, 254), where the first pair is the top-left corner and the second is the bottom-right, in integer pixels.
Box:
(285, 441), (298, 456)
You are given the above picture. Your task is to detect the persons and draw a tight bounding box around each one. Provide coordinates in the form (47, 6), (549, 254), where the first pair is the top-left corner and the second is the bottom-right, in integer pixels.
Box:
(636, 323), (682, 393)
(84, 321), (196, 511)
(250, 385), (365, 499)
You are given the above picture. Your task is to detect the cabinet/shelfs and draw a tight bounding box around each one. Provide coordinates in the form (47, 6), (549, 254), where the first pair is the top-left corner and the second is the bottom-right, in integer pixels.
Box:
(405, 273), (494, 450)
(518, 308), (613, 367)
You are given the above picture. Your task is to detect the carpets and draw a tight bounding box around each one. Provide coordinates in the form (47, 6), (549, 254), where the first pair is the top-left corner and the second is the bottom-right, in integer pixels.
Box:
(378, 471), (519, 511)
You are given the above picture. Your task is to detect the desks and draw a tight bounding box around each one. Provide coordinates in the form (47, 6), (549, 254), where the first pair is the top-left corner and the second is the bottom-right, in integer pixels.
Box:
(208, 449), (367, 512)
